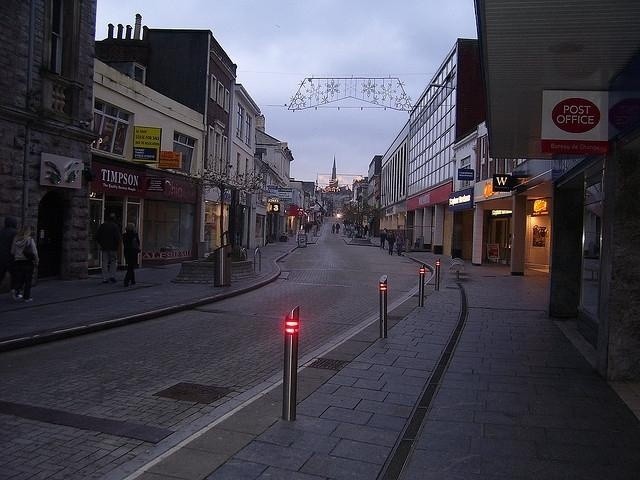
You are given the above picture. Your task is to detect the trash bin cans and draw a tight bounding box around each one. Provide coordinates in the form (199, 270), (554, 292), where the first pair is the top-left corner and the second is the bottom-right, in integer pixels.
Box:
(416, 236), (424, 251)
(214, 243), (233, 286)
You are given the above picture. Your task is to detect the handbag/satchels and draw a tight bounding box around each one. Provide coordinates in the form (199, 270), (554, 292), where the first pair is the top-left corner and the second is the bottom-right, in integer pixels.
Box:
(23, 239), (35, 261)
(129, 239), (141, 253)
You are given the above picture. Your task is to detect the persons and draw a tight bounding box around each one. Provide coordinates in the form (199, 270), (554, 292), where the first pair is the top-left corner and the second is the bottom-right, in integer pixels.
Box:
(94, 213), (121, 284)
(380, 228), (387, 250)
(386, 231), (396, 255)
(397, 232), (406, 256)
(122, 223), (140, 286)
(0, 216), (39, 303)
(332, 222), (340, 234)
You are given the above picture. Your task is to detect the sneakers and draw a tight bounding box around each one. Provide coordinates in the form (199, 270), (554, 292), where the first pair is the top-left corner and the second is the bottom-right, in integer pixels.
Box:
(8, 292), (33, 305)
(102, 277), (137, 288)
